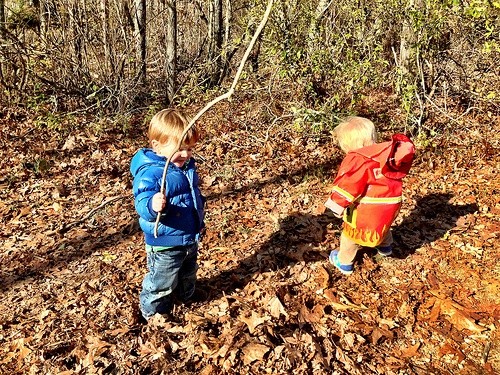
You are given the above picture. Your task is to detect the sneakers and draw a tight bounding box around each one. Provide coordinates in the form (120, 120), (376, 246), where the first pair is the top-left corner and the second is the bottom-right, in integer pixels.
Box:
(377, 246), (393, 256)
(329, 251), (354, 275)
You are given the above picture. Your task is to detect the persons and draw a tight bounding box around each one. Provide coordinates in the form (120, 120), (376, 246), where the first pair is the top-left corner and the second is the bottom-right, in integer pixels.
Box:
(324, 116), (417, 274)
(130, 108), (204, 323)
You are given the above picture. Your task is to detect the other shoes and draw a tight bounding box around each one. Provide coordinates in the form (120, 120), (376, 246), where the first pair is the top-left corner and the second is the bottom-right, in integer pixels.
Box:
(182, 288), (208, 302)
(141, 312), (171, 325)
